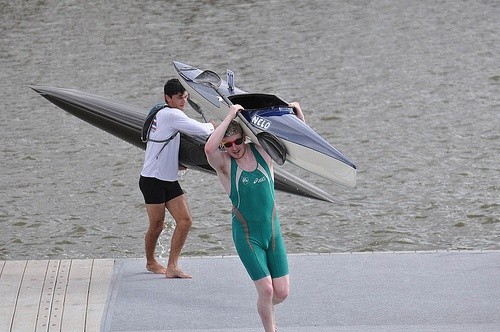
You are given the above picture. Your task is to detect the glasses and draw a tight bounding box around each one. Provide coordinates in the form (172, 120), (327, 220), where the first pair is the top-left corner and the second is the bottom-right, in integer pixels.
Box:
(222, 135), (244, 148)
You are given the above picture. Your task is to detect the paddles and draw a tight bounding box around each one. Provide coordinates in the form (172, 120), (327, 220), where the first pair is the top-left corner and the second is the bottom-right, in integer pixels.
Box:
(186, 97), (209, 125)
(192, 70), (287, 167)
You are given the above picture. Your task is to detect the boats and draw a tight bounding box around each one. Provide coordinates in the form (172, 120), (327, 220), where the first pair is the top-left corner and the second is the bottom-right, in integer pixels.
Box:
(172, 61), (356, 189)
(26, 84), (338, 203)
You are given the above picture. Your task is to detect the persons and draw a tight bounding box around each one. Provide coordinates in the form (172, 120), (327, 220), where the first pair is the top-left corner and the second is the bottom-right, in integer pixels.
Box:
(204, 102), (307, 332)
(138, 79), (216, 278)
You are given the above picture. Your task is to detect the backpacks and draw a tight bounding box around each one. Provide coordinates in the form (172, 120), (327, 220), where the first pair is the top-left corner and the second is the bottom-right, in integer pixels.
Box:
(142, 103), (179, 142)
